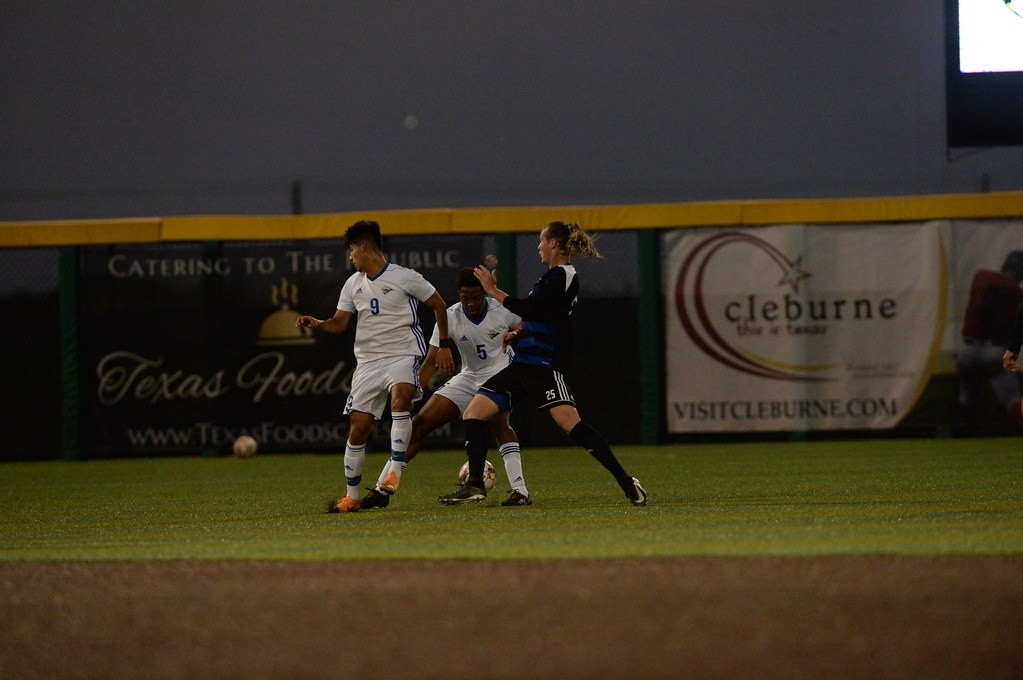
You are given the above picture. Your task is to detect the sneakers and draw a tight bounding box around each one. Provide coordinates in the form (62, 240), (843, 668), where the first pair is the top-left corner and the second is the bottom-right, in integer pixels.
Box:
(621, 475), (645, 506)
(380, 471), (400, 495)
(439, 482), (485, 503)
(500, 490), (532, 506)
(359, 488), (390, 509)
(335, 495), (361, 512)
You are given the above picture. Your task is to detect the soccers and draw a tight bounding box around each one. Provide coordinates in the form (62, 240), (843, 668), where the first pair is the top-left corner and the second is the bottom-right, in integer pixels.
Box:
(457, 458), (497, 493)
(232, 435), (259, 459)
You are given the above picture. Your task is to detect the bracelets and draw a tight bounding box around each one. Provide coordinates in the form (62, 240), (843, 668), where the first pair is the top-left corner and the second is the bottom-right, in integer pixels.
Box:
(439, 339), (450, 348)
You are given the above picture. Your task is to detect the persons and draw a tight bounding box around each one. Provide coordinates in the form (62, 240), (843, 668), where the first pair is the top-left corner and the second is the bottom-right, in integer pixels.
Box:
(353, 266), (532, 507)
(439, 222), (648, 507)
(293, 220), (455, 514)
